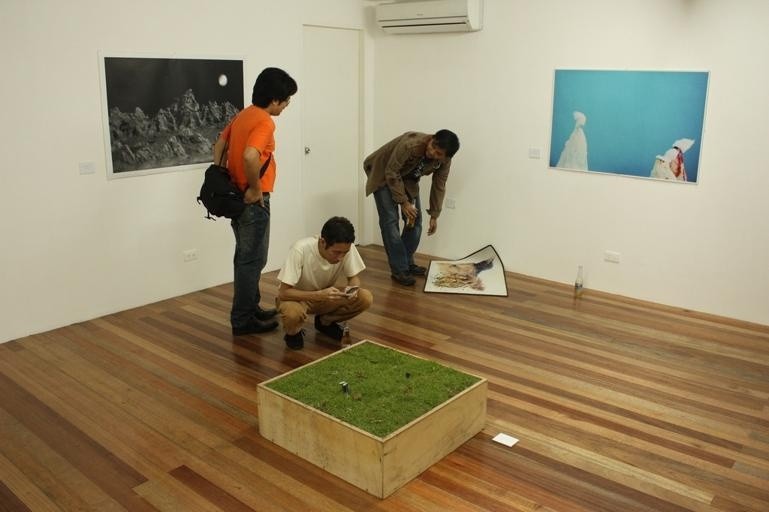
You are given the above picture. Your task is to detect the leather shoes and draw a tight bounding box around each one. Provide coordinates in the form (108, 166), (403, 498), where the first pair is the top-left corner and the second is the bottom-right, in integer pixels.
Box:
(232, 304), (279, 335)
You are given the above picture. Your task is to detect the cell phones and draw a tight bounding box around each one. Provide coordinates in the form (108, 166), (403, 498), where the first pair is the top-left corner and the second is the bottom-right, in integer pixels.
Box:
(342, 285), (359, 297)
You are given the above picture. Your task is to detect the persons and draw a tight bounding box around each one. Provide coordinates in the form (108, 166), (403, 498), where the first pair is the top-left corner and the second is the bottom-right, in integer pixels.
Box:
(363, 128), (461, 286)
(195, 67), (298, 337)
(275, 217), (372, 348)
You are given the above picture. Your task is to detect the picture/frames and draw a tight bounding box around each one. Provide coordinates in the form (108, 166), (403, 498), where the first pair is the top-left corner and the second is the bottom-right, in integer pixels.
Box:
(99, 52), (249, 181)
(547, 67), (712, 187)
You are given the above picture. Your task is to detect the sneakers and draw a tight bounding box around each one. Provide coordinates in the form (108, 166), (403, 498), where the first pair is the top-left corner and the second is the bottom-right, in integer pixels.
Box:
(285, 332), (303, 349)
(315, 315), (343, 340)
(392, 273), (415, 285)
(410, 265), (426, 274)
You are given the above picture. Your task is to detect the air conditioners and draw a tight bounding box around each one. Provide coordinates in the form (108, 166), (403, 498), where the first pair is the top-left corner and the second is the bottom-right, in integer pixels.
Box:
(377, 0), (483, 36)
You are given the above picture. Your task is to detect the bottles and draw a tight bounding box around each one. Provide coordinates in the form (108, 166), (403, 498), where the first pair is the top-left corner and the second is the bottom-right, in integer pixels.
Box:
(341, 321), (352, 349)
(406, 198), (416, 228)
(574, 266), (583, 298)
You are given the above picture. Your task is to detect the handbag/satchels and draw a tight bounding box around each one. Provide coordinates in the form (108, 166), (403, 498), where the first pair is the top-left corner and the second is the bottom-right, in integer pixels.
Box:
(201, 165), (245, 219)
